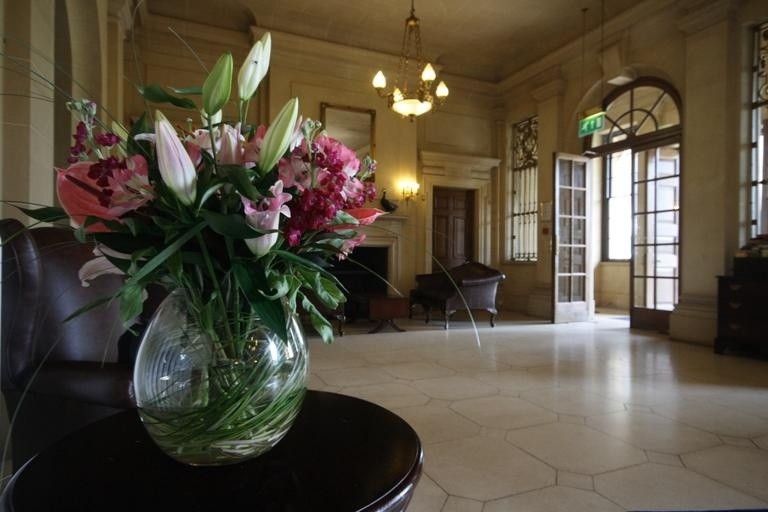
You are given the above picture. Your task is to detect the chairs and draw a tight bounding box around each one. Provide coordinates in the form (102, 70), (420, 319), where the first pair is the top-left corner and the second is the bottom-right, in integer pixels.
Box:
(1, 217), (174, 472)
(408, 260), (507, 330)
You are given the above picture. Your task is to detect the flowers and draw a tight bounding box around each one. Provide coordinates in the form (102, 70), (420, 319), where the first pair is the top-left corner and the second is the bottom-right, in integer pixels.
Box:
(0, 25), (479, 485)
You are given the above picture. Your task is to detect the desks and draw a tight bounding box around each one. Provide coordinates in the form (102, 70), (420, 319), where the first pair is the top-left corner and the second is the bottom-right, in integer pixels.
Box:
(0, 386), (426, 511)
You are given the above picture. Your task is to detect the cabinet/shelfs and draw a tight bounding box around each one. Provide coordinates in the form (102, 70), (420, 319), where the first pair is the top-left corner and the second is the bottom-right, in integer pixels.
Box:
(713, 274), (768, 361)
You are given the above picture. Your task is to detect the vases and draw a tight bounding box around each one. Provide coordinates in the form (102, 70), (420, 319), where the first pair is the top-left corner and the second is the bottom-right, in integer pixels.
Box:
(132, 285), (311, 468)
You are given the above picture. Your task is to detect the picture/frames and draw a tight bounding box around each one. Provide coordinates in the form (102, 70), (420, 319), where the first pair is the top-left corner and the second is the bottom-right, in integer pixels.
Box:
(319, 101), (377, 184)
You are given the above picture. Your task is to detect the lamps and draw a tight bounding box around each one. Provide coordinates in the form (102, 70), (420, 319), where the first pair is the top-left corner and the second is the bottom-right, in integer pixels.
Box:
(373, 2), (450, 118)
